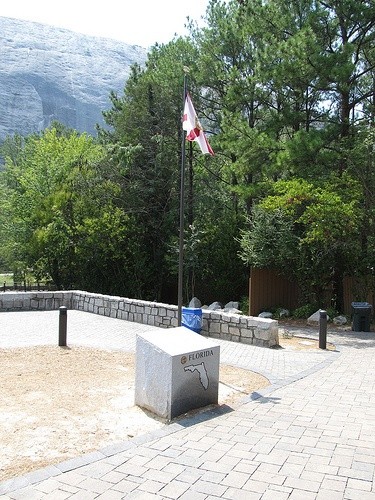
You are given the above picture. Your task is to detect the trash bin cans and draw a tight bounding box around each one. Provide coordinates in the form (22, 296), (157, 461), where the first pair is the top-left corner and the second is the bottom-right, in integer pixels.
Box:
(350, 301), (373, 333)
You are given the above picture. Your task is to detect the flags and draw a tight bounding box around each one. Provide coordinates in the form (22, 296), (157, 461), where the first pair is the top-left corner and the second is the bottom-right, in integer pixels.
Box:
(182, 93), (214, 156)
